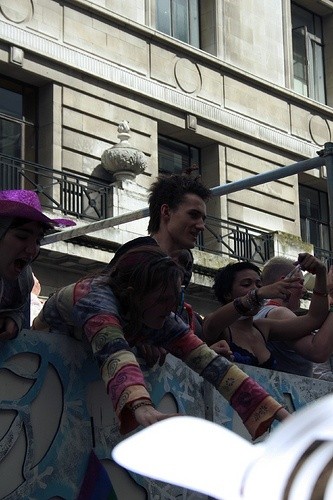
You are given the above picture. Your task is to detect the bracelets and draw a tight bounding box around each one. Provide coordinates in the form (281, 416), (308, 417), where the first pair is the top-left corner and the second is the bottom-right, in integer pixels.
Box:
(132, 400), (151, 411)
(233, 288), (264, 317)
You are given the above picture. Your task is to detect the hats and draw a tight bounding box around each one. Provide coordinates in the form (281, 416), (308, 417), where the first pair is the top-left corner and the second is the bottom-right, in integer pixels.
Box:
(0, 188), (77, 226)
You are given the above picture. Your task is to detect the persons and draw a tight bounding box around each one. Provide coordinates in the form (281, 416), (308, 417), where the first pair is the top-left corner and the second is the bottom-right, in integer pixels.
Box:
(250, 250), (333, 365)
(198, 250), (327, 373)
(30, 247), (292, 437)
(324, 258), (333, 310)
(0, 187), (59, 345)
(102, 170), (212, 290)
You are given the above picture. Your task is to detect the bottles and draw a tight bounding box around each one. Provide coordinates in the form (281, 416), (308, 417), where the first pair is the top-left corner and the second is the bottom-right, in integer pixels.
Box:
(280, 263), (301, 303)
